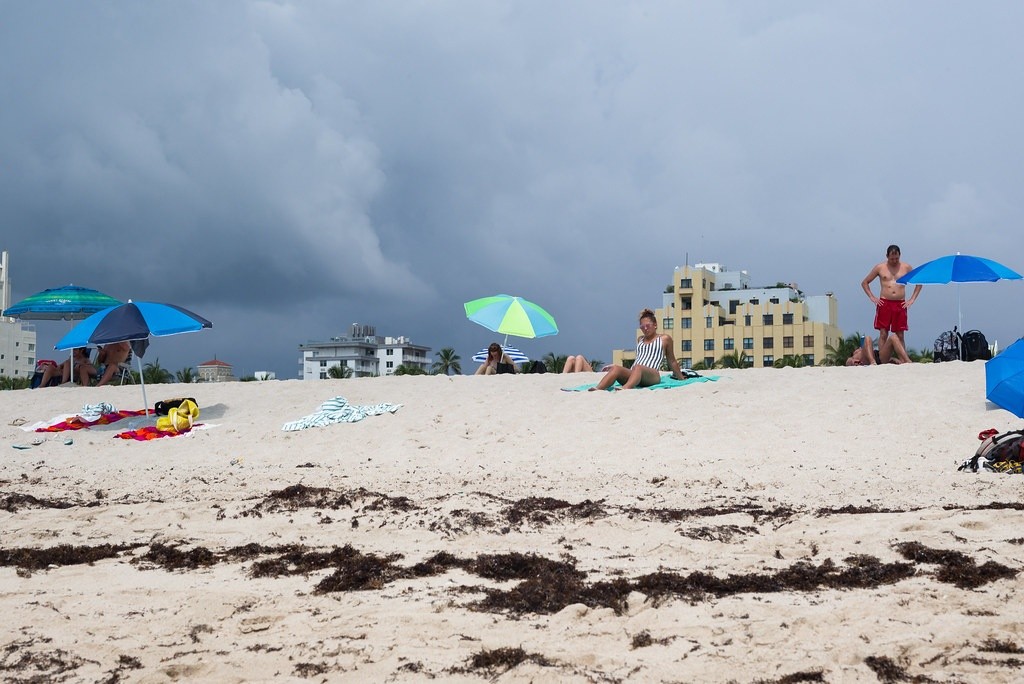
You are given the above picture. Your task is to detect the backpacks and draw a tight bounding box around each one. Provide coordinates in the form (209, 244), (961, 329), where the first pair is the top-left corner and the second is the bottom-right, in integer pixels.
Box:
(962, 330), (991, 361)
(934, 325), (963, 363)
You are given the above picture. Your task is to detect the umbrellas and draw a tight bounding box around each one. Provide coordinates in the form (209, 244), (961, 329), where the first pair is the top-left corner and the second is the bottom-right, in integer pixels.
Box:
(985, 338), (1024, 418)
(896, 251), (1024, 361)
(2, 283), (126, 383)
(52, 299), (214, 418)
(464, 294), (559, 363)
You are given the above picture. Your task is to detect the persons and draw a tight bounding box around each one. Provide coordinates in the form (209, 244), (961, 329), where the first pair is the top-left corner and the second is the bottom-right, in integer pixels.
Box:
(861, 245), (922, 363)
(588, 309), (684, 391)
(80, 341), (130, 387)
(563, 355), (593, 373)
(474, 343), (515, 375)
(846, 333), (912, 365)
(37, 347), (91, 388)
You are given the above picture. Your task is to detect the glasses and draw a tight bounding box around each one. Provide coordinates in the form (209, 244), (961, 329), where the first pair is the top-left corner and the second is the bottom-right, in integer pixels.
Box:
(640, 323), (653, 329)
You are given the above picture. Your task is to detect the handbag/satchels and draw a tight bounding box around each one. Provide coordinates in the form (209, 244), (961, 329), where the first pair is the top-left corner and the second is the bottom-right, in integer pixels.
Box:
(156, 400), (199, 433)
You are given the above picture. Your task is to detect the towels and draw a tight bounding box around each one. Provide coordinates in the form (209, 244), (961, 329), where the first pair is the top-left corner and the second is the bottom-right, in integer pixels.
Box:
(282, 394), (406, 432)
(113, 424), (207, 441)
(561, 367), (721, 391)
(17, 401), (155, 436)
